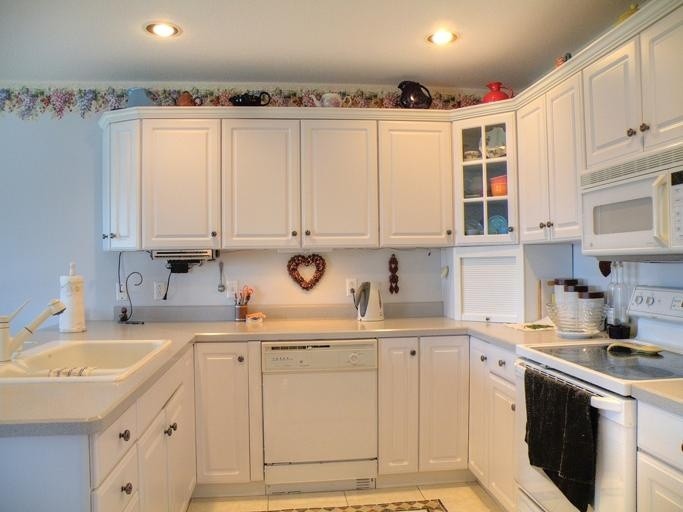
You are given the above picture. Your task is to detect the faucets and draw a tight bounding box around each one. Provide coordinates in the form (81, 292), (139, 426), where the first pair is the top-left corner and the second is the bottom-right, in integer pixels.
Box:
(0, 300), (66, 362)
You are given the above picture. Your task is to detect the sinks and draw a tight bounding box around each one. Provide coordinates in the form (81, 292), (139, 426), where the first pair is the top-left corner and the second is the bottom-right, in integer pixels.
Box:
(0, 338), (172, 383)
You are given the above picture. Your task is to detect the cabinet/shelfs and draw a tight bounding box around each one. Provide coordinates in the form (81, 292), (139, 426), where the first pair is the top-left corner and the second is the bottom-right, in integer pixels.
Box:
(378, 110), (519, 247)
(0, 403), (139, 511)
(637, 400), (683, 512)
(220, 118), (378, 251)
(193, 341), (250, 486)
(101, 117), (142, 252)
(375, 334), (518, 509)
(142, 119), (220, 251)
(135, 345), (196, 511)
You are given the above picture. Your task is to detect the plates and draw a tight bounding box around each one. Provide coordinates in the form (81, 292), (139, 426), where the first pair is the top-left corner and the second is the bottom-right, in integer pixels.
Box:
(465, 229), (480, 235)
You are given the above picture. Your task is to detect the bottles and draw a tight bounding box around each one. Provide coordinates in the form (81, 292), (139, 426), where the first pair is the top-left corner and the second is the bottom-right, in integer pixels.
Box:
(564, 283), (587, 302)
(605, 260), (630, 338)
(552, 277), (577, 302)
(579, 290), (605, 307)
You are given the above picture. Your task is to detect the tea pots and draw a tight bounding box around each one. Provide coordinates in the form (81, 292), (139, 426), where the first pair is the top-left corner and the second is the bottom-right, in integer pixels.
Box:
(126, 86), (158, 107)
(307, 92), (353, 107)
(167, 92), (203, 106)
(396, 80), (433, 110)
(227, 92), (270, 106)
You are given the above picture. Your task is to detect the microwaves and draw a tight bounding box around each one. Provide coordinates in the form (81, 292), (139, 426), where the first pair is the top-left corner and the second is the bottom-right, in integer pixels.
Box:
(578, 166), (683, 258)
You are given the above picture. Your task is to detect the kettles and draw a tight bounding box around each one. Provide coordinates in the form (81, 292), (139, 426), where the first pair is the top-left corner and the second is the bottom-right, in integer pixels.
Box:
(354, 280), (383, 322)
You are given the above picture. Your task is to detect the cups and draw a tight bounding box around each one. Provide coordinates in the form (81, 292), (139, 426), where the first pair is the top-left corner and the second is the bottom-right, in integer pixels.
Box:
(234, 305), (249, 321)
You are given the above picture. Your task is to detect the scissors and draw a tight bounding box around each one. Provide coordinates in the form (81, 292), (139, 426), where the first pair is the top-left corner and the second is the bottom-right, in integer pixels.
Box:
(242, 286), (253, 298)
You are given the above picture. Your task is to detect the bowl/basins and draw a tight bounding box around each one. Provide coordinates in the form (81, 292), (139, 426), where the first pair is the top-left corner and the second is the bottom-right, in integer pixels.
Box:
(544, 302), (609, 333)
(488, 174), (507, 197)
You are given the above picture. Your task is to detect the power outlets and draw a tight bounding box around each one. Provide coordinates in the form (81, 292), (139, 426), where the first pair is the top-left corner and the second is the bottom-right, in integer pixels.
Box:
(115, 283), (129, 301)
(346, 278), (357, 296)
(152, 280), (166, 300)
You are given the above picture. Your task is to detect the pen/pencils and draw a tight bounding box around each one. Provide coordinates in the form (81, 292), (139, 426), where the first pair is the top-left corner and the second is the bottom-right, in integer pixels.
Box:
(233, 293), (249, 306)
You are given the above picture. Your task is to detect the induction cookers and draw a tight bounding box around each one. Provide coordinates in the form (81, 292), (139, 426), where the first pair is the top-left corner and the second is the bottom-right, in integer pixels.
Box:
(513, 338), (683, 396)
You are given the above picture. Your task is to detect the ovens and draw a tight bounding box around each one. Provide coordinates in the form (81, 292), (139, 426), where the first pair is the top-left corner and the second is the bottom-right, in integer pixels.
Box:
(504, 361), (637, 512)
